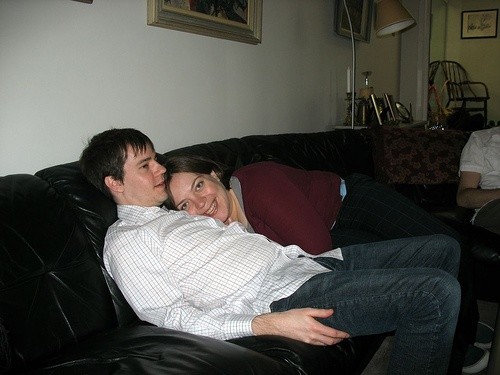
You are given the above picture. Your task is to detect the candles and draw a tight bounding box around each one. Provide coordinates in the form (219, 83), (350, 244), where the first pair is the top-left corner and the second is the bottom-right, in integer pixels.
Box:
(347, 66), (350, 93)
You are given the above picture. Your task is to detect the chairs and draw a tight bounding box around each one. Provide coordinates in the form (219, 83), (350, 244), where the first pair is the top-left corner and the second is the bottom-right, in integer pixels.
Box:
(427, 60), (490, 131)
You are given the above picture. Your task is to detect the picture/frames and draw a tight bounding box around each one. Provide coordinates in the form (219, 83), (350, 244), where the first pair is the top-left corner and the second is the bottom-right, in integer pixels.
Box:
(147, 0), (264, 45)
(333, 0), (374, 44)
(461, 9), (499, 39)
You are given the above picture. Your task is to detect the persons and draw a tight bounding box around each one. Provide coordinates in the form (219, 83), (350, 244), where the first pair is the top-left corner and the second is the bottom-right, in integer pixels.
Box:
(457, 126), (500, 233)
(163, 156), (493, 375)
(81, 128), (462, 375)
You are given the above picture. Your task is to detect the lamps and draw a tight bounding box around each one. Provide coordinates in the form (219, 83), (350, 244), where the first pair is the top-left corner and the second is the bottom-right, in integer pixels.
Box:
(374, 0), (417, 39)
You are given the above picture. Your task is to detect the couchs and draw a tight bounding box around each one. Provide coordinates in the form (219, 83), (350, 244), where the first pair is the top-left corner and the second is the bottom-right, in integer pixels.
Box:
(0, 122), (500, 375)
